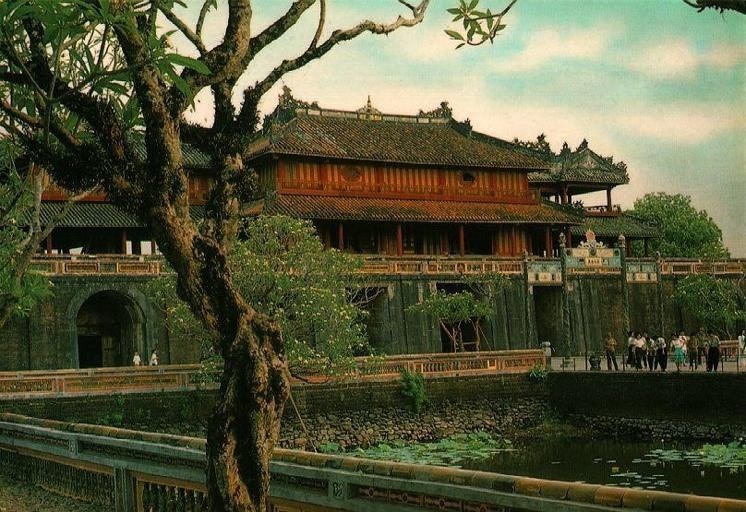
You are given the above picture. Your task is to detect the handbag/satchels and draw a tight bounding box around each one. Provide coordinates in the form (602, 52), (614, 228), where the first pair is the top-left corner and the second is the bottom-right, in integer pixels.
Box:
(671, 345), (675, 352)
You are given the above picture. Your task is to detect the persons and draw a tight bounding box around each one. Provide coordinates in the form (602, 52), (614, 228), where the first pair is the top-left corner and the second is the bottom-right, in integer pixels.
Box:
(149, 349), (159, 366)
(603, 331), (619, 372)
(738, 328), (745, 356)
(132, 351), (141, 366)
(625, 327), (722, 371)
